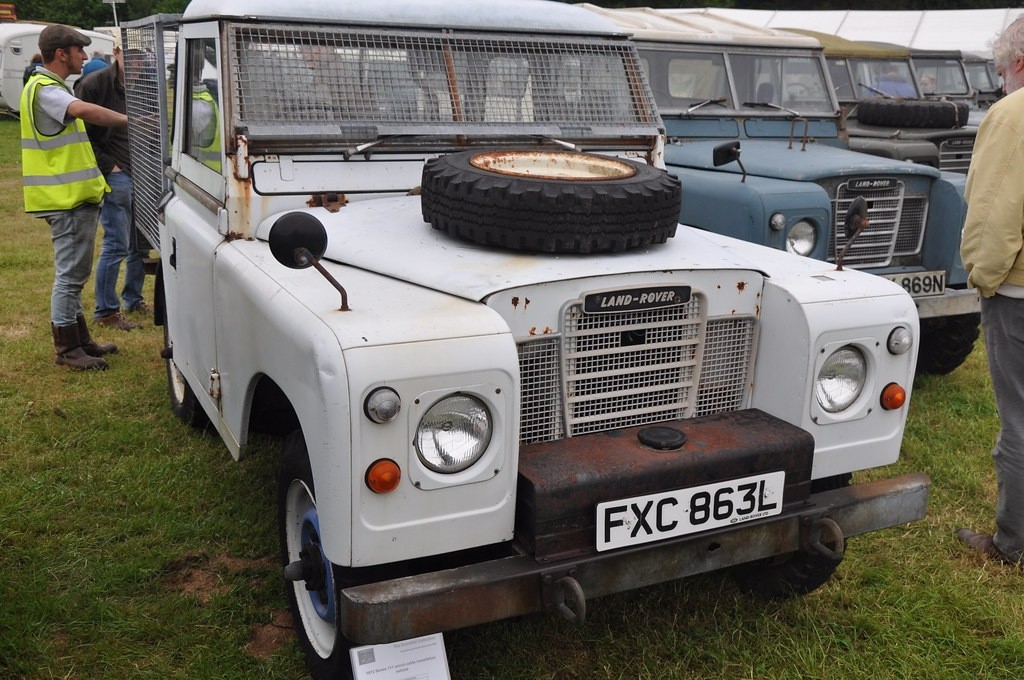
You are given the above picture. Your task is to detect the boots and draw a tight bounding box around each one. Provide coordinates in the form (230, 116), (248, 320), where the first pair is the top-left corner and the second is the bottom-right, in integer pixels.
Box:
(51, 321), (109, 371)
(76, 315), (118, 358)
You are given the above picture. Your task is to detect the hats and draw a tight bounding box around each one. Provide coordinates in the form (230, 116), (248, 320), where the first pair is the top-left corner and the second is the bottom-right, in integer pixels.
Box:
(38, 25), (91, 51)
(112, 29), (146, 52)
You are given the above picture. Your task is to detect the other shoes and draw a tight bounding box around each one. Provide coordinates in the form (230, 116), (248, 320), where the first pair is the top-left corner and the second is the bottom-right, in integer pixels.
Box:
(958, 529), (1013, 565)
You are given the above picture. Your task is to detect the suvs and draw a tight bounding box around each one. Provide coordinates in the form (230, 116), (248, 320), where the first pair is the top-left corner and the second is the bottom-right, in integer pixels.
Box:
(117, 0), (935, 680)
(0, 0), (1010, 379)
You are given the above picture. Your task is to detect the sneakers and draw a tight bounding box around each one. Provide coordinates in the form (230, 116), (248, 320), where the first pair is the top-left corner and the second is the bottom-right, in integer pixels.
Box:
(93, 312), (144, 332)
(125, 302), (154, 315)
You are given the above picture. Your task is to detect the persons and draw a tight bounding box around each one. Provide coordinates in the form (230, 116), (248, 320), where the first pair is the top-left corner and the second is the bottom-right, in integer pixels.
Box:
(244, 42), (380, 121)
(189, 48), (222, 174)
(955, 13), (1024, 577)
(866, 62), (938, 98)
(20, 24), (174, 371)
